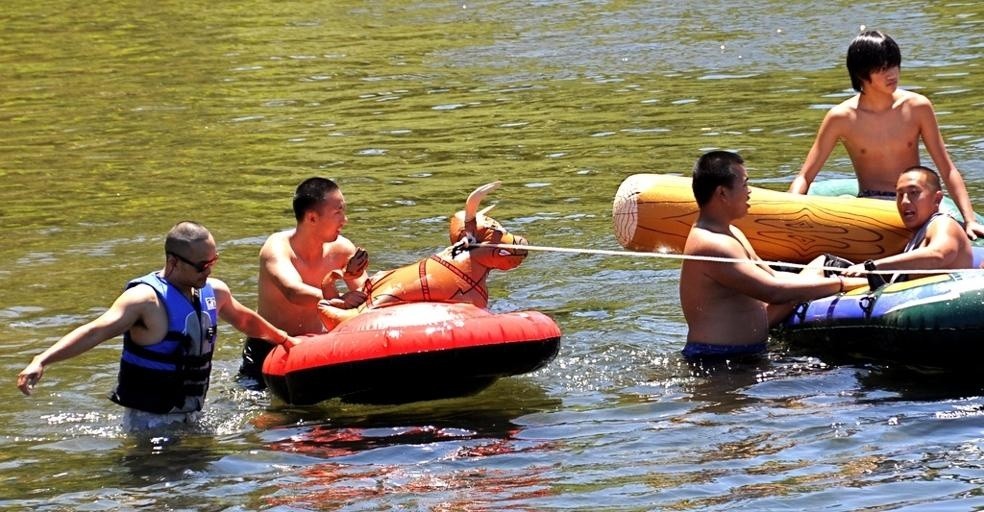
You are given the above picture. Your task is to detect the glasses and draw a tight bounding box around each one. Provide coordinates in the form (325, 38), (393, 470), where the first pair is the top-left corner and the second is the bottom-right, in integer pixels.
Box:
(165, 250), (220, 274)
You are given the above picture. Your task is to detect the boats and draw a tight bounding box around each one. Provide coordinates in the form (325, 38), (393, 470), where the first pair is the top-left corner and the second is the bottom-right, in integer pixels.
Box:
(262, 302), (562, 406)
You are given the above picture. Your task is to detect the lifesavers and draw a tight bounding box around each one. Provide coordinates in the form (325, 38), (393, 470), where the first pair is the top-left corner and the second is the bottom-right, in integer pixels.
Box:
(805, 179), (984, 248)
(772, 269), (984, 365)
(262, 182), (561, 404)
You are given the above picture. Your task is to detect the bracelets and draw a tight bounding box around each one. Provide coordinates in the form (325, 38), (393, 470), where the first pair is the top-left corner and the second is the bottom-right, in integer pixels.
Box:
(837, 275), (844, 292)
(278, 332), (288, 346)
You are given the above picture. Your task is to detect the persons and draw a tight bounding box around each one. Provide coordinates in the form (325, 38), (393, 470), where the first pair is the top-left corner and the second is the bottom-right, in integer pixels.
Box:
(230, 176), (369, 392)
(679, 150), (869, 370)
(787, 31), (984, 241)
(17, 220), (302, 431)
(840, 165), (973, 281)
